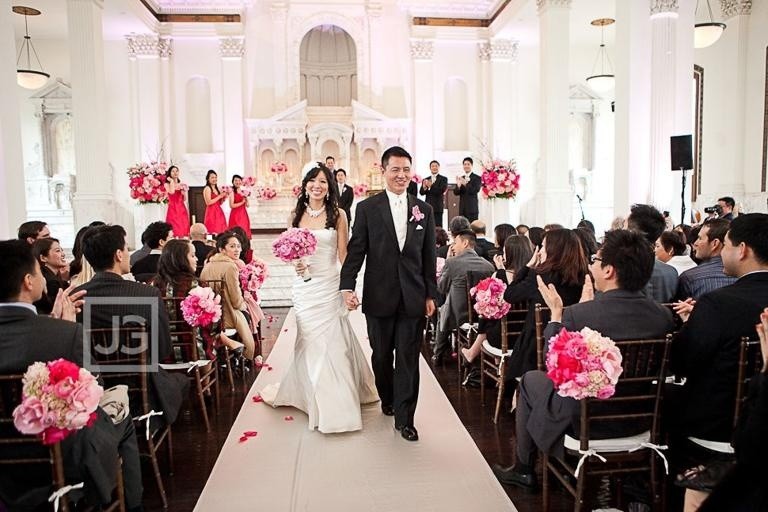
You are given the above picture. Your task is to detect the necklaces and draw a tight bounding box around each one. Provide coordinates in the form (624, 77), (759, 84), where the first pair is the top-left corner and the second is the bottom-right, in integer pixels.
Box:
(305, 203), (325, 218)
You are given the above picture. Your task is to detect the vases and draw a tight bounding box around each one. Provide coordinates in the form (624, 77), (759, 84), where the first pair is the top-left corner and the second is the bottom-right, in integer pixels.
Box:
(491, 197), (510, 230)
(133, 205), (169, 250)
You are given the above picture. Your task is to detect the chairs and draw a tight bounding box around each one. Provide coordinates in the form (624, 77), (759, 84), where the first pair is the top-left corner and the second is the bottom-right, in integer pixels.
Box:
(406, 268), (768, 512)
(0, 257), (264, 510)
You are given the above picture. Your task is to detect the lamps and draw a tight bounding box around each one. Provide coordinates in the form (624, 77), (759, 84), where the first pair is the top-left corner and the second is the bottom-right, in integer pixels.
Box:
(583, 14), (619, 101)
(11, 4), (55, 88)
(689, 0), (725, 52)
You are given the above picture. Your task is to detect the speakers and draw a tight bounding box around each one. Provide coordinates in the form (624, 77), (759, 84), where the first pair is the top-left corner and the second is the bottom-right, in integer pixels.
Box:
(671, 134), (692, 170)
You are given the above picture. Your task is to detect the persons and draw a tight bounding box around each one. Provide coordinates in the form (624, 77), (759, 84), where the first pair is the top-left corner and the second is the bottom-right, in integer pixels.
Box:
(203, 170), (227, 235)
(229, 175), (252, 238)
(164, 166), (190, 237)
(257, 162), (381, 434)
(407, 181), (417, 198)
(453, 157), (481, 223)
(420, 160), (447, 227)
(338, 146), (437, 441)
(335, 168), (354, 232)
(326, 157), (337, 182)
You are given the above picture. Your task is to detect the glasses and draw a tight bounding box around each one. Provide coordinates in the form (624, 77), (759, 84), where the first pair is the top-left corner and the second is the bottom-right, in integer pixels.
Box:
(590, 252), (602, 263)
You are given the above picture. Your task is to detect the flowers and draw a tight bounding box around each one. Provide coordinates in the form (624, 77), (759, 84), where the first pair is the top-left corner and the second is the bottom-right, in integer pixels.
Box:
(126, 138), (182, 204)
(269, 225), (321, 282)
(217, 157), (427, 200)
(470, 136), (520, 202)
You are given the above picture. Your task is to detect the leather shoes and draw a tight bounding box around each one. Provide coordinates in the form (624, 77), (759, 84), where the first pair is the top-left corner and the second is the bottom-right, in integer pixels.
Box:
(381, 401), (396, 415)
(431, 355), (442, 367)
(136, 451), (171, 476)
(395, 421), (419, 441)
(493, 462), (539, 492)
(459, 343), (472, 368)
(557, 474), (575, 497)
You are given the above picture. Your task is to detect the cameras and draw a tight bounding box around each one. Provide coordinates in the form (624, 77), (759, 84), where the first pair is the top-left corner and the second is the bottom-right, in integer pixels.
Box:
(705, 205), (722, 215)
